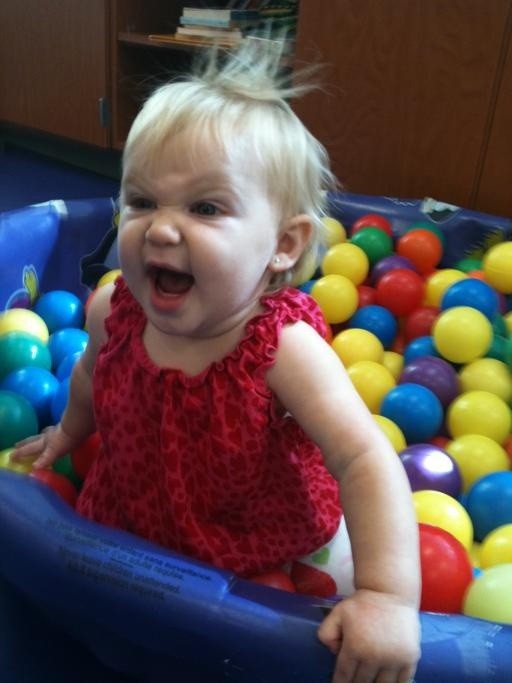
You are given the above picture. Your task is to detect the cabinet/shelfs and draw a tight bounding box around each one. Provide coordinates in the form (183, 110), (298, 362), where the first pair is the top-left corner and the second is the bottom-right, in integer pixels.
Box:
(0, 0), (109, 180)
(109, 0), (301, 182)
(293, 1), (512, 211)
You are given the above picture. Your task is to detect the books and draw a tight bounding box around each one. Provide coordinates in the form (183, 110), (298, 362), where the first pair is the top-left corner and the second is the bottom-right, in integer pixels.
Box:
(148, 6), (295, 57)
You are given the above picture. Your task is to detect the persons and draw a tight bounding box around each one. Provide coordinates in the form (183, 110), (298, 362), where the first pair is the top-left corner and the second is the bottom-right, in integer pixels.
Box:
(8, 17), (423, 681)
